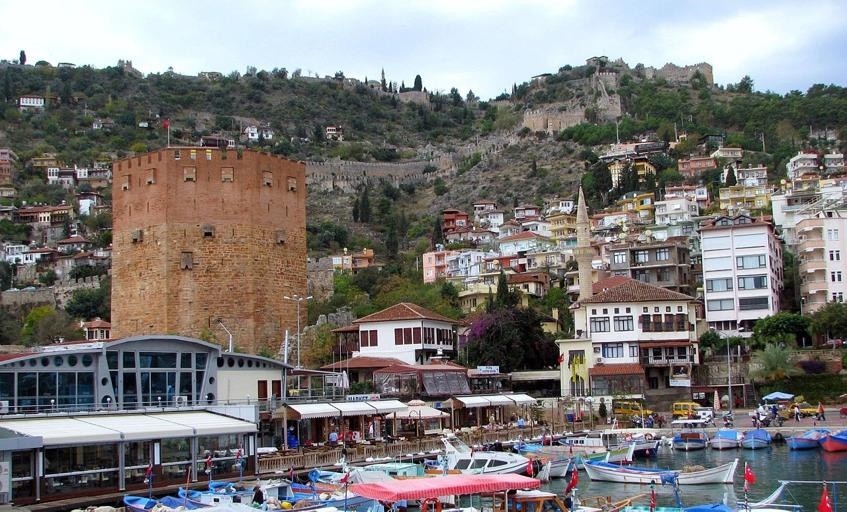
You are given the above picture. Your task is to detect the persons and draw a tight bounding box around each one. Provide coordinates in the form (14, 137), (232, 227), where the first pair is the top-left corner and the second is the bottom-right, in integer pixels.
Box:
(517, 416), (526, 429)
(251, 486), (263, 505)
(758, 403), (765, 413)
(599, 397), (608, 425)
(236, 450), (243, 459)
(816, 401), (825, 421)
(489, 440), (503, 451)
(772, 405), (778, 419)
(329, 430), (338, 449)
(489, 414), (495, 431)
(794, 405), (800, 422)
(144, 477), (149, 488)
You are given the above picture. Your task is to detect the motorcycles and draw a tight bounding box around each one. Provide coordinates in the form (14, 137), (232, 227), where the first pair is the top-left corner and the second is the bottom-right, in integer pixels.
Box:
(632, 411), (784, 428)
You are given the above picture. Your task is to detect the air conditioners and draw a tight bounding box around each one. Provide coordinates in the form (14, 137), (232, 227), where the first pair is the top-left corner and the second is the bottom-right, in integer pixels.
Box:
(0, 462), (9, 474)
(597, 357), (604, 363)
(0, 401), (9, 413)
(0, 474), (10, 492)
(173, 396), (187, 407)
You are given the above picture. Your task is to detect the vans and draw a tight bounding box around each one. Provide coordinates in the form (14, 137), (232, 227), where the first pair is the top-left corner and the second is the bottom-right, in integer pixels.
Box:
(613, 402), (654, 419)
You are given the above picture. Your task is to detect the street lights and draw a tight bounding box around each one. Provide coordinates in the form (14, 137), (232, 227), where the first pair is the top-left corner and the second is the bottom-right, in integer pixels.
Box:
(211, 314), (233, 353)
(284, 294), (314, 390)
(710, 326), (745, 412)
(327, 393), (597, 456)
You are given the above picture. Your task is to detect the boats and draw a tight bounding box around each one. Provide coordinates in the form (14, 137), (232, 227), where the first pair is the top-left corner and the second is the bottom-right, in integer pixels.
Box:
(784, 428), (833, 450)
(710, 429), (744, 450)
(741, 428), (773, 450)
(671, 429), (710, 451)
(818, 430), (847, 452)
(119, 426), (662, 512)
(69, 505), (118, 512)
(581, 458), (739, 485)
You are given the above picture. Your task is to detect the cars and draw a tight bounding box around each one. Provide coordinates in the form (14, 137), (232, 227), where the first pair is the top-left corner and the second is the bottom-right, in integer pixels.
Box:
(821, 339), (843, 348)
(672, 401), (819, 421)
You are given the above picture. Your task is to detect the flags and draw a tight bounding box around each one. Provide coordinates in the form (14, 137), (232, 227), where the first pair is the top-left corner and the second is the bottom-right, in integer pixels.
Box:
(744, 464), (757, 485)
(288, 467), (293, 478)
(145, 464), (152, 476)
(207, 457), (213, 468)
(817, 487), (833, 512)
(338, 472), (350, 483)
(557, 351), (586, 382)
(527, 460), (533, 475)
(564, 468), (578, 496)
(162, 118), (170, 127)
